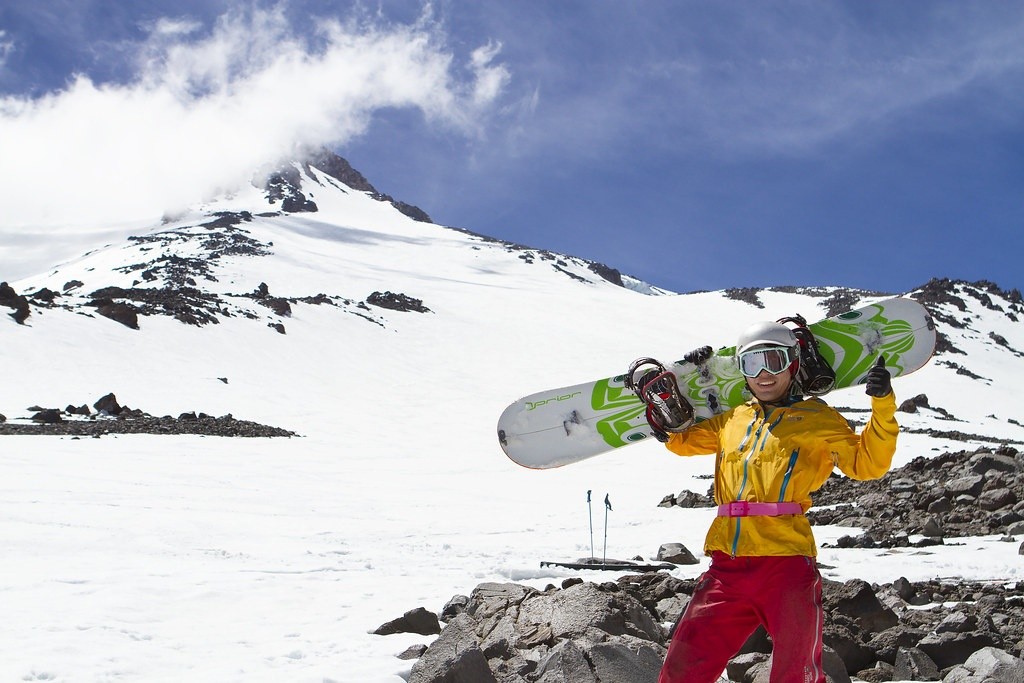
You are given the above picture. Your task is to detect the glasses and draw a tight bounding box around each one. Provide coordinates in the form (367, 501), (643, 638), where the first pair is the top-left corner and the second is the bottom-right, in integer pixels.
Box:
(736, 342), (801, 378)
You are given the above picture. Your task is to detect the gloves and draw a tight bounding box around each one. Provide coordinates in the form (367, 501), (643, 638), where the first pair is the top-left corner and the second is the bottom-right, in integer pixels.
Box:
(684, 345), (727, 365)
(865, 355), (892, 397)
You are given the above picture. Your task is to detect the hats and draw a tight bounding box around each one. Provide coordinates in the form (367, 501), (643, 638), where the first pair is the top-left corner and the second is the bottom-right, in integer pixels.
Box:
(736, 321), (799, 357)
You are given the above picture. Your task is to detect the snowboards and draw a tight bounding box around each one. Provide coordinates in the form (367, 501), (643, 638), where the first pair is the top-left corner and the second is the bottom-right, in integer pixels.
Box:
(496, 297), (938, 471)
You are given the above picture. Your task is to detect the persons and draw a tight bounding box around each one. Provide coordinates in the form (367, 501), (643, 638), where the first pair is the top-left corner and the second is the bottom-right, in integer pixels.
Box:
(654, 319), (898, 683)
